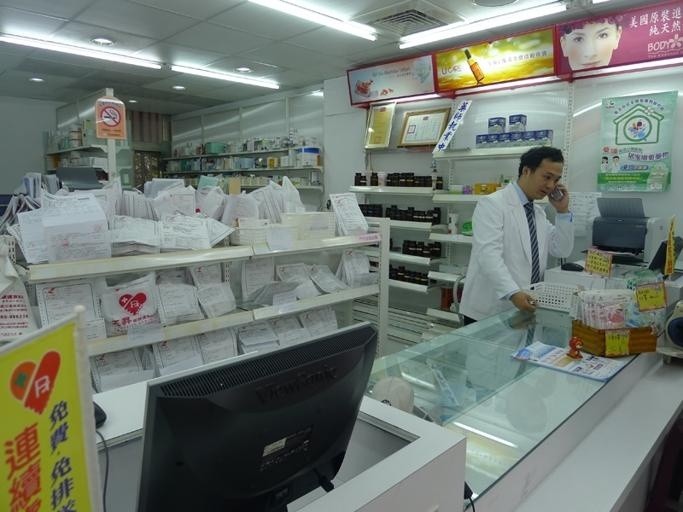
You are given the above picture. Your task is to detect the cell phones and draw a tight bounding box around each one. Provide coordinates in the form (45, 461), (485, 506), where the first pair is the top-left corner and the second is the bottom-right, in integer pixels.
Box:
(552, 186), (563, 201)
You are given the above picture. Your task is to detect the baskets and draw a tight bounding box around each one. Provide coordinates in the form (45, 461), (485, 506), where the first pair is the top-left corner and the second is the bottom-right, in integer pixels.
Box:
(526, 281), (582, 312)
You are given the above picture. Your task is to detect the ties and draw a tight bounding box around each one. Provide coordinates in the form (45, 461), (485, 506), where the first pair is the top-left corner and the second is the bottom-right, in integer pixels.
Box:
(514, 325), (536, 379)
(523, 202), (539, 289)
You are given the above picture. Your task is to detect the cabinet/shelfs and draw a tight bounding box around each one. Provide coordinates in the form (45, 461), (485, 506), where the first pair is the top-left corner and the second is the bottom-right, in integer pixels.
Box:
(349, 182), (440, 296)
(164, 143), (325, 193)
(45, 142), (134, 191)
(19, 217), (390, 392)
(426, 144), (554, 324)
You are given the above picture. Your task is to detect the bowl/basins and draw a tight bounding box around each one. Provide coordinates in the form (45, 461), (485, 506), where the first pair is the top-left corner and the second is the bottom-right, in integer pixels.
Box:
(238, 157), (252, 170)
(205, 141), (226, 154)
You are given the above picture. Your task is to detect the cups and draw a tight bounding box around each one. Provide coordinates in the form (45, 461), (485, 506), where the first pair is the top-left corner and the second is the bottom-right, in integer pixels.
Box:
(448, 210), (459, 235)
(355, 81), (369, 97)
(447, 173), (511, 196)
(304, 172), (312, 184)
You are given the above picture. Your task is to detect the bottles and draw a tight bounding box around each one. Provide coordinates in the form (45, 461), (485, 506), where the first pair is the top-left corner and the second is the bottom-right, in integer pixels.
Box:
(464, 49), (484, 82)
(354, 171), (443, 189)
(401, 239), (440, 258)
(388, 264), (437, 286)
(358, 203), (442, 226)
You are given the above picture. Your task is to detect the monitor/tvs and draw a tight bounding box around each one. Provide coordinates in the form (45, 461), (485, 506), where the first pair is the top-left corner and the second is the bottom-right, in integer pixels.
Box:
(134, 321), (379, 512)
(648, 235), (683, 280)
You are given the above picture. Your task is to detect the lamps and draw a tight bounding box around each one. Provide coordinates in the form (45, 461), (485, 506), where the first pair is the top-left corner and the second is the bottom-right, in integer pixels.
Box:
(169, 64), (280, 91)
(250, 0), (378, 42)
(0, 32), (165, 69)
(397, 4), (572, 50)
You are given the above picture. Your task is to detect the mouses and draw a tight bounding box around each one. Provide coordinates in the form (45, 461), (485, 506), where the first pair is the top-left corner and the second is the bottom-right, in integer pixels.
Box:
(561, 263), (584, 271)
(92, 401), (107, 428)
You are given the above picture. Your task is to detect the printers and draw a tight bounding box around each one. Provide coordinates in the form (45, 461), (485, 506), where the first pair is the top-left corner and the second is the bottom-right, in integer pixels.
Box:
(581, 198), (663, 267)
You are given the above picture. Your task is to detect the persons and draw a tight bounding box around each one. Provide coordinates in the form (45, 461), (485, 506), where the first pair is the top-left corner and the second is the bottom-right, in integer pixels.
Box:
(558, 15), (624, 72)
(599, 157), (608, 172)
(457, 145), (576, 402)
(611, 156), (620, 172)
